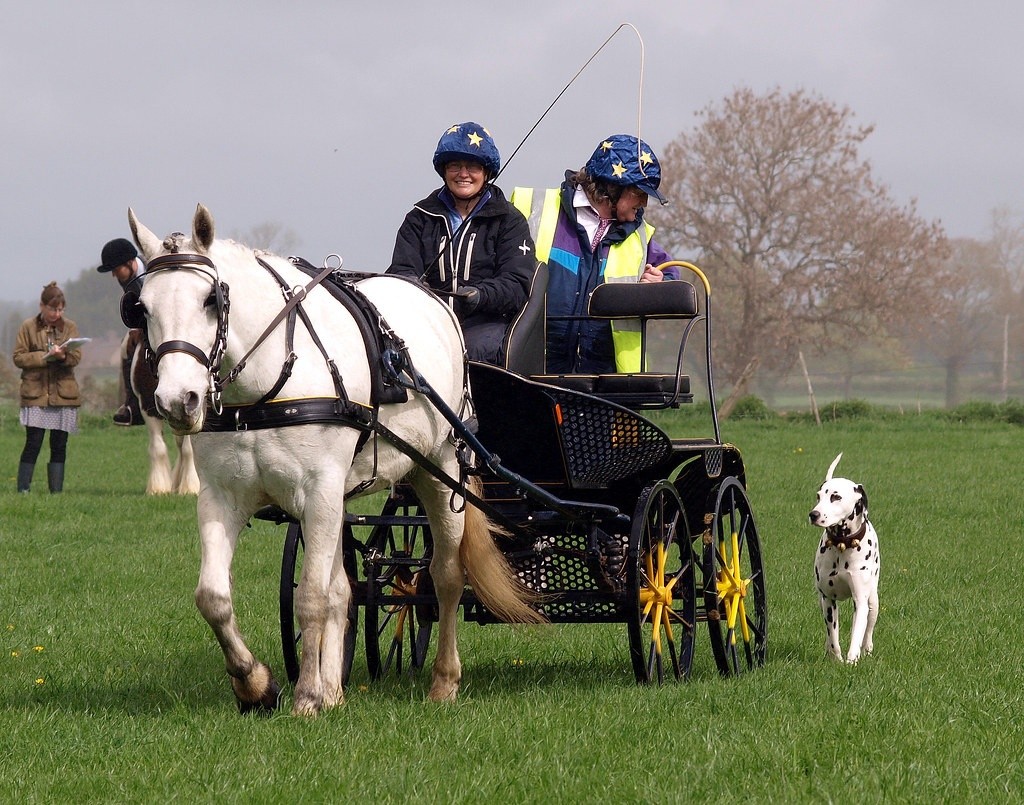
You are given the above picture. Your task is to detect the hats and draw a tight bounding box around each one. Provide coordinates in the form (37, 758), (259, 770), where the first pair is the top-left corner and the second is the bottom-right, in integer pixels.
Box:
(432, 121), (500, 180)
(97, 237), (138, 272)
(586, 133), (667, 200)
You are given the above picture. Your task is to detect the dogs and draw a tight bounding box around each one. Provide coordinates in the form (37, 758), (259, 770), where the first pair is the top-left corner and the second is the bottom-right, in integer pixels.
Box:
(809, 451), (880, 664)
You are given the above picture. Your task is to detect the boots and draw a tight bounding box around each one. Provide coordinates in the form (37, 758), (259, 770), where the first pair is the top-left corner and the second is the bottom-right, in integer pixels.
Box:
(18, 461), (34, 492)
(112, 359), (145, 424)
(46, 461), (65, 494)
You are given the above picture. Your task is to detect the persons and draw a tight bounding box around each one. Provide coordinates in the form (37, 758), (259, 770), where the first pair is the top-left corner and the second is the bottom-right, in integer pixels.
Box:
(96, 238), (148, 426)
(12, 281), (82, 495)
(512, 135), (680, 375)
(385, 122), (536, 469)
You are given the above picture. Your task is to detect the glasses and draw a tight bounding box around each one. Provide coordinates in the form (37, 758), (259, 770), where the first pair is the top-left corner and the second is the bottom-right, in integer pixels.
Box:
(444, 159), (483, 172)
(44, 304), (64, 312)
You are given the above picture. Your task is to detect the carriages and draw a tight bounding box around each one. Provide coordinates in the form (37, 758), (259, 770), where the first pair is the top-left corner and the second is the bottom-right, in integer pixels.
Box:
(125, 201), (767, 717)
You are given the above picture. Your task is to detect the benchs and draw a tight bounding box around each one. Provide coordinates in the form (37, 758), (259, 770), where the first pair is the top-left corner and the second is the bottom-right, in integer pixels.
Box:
(499, 260), (705, 409)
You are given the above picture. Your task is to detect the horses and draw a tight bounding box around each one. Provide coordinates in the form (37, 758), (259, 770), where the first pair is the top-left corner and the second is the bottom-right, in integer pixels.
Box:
(115, 204), (560, 720)
